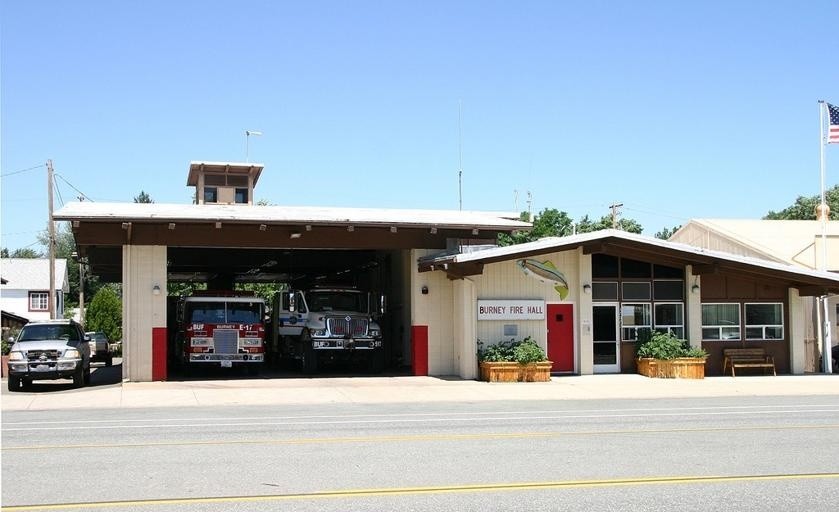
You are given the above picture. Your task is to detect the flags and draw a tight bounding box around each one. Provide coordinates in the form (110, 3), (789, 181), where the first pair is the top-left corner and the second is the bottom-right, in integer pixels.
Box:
(827, 103), (838, 145)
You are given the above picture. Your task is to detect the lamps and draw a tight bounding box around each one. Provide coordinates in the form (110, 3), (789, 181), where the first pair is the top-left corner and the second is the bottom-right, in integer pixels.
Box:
(153, 285), (160, 295)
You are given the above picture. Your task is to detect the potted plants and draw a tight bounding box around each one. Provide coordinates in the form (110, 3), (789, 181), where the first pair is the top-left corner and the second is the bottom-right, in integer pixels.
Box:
(634, 331), (711, 379)
(477, 336), (554, 382)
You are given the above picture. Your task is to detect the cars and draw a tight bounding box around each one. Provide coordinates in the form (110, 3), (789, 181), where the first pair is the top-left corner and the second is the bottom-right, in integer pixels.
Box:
(7, 318), (112, 392)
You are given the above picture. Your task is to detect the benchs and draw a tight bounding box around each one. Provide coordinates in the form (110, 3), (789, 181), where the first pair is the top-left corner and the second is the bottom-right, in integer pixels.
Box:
(722, 348), (777, 376)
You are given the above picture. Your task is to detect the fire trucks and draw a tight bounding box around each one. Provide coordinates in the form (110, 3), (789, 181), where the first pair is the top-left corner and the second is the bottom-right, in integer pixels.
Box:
(171, 285), (389, 376)
